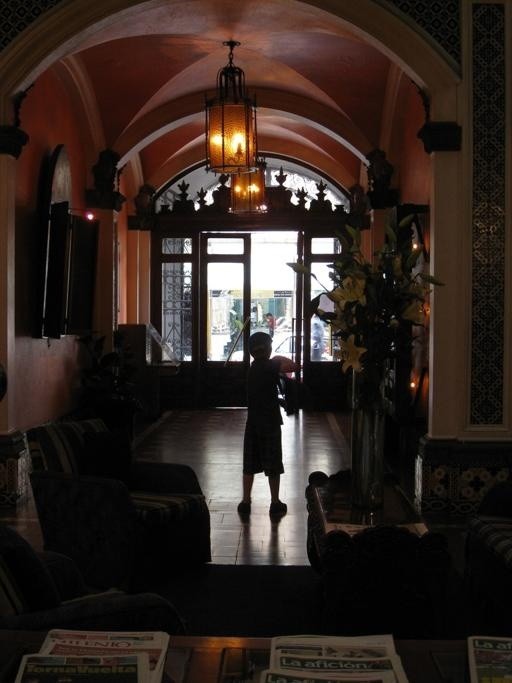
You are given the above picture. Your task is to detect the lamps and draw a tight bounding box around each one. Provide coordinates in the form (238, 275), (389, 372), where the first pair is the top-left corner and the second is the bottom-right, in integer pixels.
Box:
(202, 34), (271, 219)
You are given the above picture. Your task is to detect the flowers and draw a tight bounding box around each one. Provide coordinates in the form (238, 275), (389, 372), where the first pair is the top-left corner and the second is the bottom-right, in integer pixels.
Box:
(295, 209), (445, 519)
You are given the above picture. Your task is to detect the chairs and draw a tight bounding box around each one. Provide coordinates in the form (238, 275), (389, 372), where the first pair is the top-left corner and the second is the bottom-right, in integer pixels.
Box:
(38, 422), (214, 590)
(2, 516), (191, 633)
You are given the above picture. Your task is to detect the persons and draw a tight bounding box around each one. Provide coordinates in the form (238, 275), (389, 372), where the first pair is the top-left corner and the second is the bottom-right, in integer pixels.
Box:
(238, 332), (287, 517)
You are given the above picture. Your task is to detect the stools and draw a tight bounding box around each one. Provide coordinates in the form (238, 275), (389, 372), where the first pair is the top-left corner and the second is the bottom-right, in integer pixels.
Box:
(465, 474), (510, 607)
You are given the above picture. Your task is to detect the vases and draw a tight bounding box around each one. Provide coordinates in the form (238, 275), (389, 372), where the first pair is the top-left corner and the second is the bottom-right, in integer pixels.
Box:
(344, 361), (394, 508)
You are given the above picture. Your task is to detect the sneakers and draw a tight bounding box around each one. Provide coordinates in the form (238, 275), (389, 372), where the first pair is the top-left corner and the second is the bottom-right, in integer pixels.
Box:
(270, 500), (287, 517)
(238, 503), (251, 516)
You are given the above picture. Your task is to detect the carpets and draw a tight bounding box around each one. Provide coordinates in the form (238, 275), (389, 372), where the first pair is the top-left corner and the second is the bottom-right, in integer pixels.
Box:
(171, 562), (322, 634)
